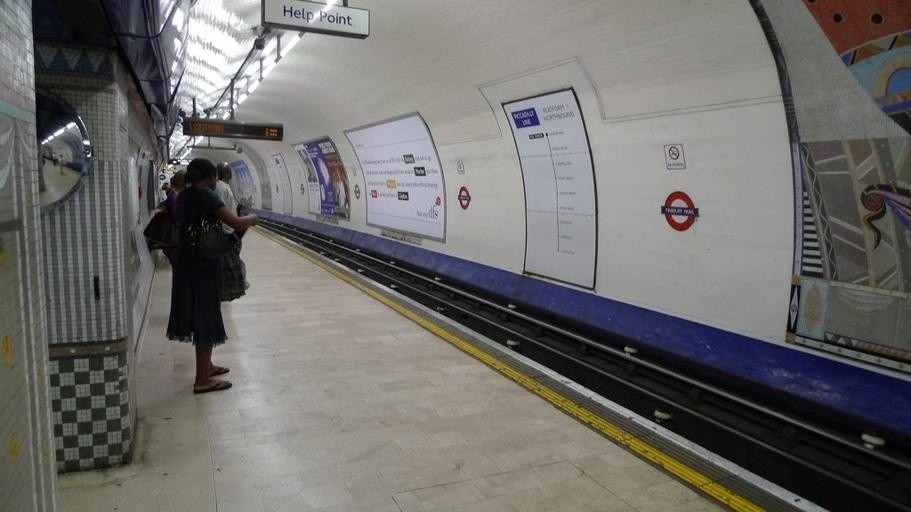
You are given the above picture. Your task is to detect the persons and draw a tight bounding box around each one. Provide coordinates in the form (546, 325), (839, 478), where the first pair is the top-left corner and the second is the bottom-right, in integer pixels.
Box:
(163, 170), (186, 218)
(58, 154), (65, 174)
(158, 183), (168, 202)
(335, 168), (348, 207)
(51, 151), (56, 166)
(213, 161), (239, 244)
(165, 157), (260, 395)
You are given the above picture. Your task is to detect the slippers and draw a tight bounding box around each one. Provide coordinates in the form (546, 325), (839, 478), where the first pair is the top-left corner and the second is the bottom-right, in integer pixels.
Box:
(210, 366), (230, 377)
(193, 379), (232, 393)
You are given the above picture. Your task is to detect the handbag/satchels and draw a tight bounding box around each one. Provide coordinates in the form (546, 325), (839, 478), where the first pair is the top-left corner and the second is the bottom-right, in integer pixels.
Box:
(220, 225), (250, 300)
(144, 210), (180, 249)
(181, 184), (228, 261)
